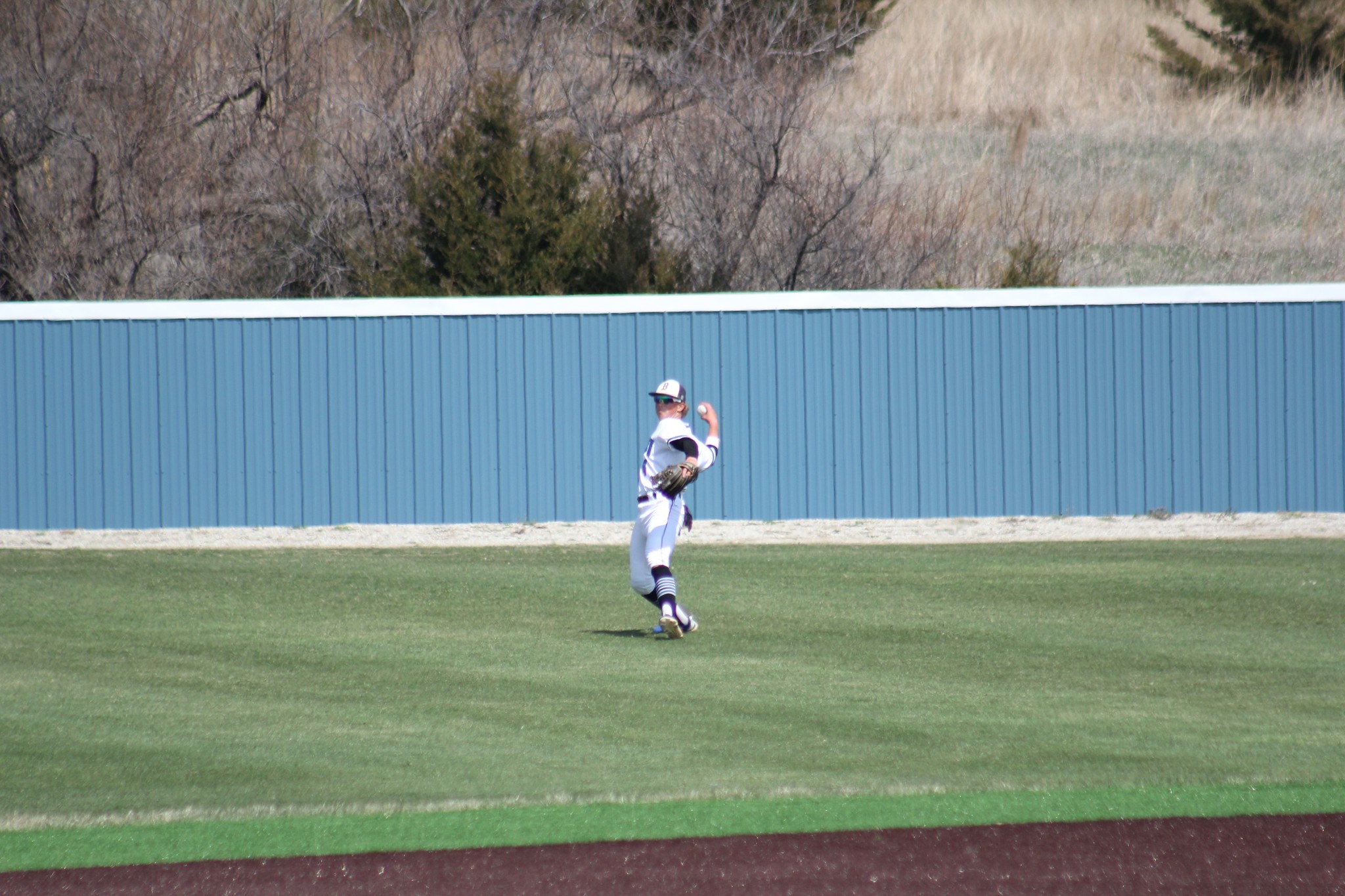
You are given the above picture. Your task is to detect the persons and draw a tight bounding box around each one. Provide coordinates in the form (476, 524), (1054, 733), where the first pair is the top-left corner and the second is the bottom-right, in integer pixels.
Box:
(630, 380), (719, 637)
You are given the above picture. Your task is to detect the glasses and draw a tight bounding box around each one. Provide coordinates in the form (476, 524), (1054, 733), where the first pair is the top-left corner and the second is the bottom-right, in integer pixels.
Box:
(654, 396), (676, 404)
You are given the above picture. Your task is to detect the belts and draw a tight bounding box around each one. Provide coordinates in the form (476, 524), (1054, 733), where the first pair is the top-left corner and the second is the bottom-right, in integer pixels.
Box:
(636, 493), (656, 504)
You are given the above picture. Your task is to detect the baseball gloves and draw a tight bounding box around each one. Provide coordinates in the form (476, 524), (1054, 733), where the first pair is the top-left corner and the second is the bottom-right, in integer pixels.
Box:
(650, 461), (699, 501)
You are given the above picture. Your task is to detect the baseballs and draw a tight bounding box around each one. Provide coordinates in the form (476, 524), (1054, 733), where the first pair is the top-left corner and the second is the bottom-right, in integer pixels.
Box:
(696, 404), (707, 416)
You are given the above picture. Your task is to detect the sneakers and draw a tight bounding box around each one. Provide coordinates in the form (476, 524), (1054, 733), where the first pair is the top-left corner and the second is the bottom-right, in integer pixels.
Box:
(679, 616), (698, 633)
(659, 617), (683, 639)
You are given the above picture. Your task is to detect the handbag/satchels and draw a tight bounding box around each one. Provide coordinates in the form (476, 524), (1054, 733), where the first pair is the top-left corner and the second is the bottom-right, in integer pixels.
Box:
(683, 505), (693, 532)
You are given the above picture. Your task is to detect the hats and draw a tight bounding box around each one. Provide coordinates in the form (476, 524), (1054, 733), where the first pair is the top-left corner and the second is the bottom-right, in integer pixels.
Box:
(649, 379), (686, 402)
